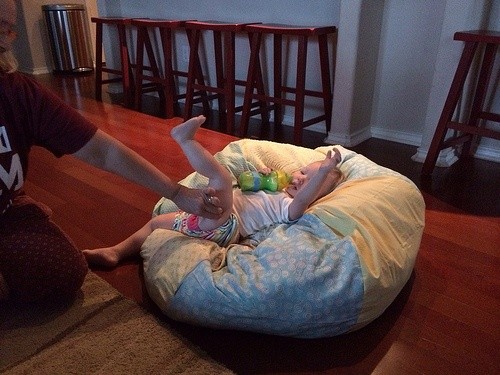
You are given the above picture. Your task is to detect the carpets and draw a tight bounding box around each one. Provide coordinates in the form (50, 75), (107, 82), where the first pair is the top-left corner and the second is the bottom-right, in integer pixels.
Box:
(0, 266), (237, 375)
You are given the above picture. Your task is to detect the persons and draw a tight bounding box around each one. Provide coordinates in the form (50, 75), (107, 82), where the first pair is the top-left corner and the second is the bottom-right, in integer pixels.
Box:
(0, 1), (224, 321)
(80, 114), (344, 268)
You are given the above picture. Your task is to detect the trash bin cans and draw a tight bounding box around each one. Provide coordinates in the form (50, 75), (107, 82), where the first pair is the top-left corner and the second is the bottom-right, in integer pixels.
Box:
(41, 4), (94, 77)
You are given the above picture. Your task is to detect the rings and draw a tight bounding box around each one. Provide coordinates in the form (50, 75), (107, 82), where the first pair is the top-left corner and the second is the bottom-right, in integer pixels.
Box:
(209, 196), (213, 203)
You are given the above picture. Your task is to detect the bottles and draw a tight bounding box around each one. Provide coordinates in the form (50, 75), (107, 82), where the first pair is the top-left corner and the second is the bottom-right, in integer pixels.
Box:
(237, 168), (294, 189)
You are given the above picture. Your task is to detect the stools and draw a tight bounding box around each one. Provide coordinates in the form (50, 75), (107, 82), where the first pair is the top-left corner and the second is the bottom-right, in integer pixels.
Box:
(89, 15), (166, 111)
(130, 17), (210, 118)
(237, 21), (336, 147)
(419, 27), (500, 176)
(178, 20), (271, 137)
